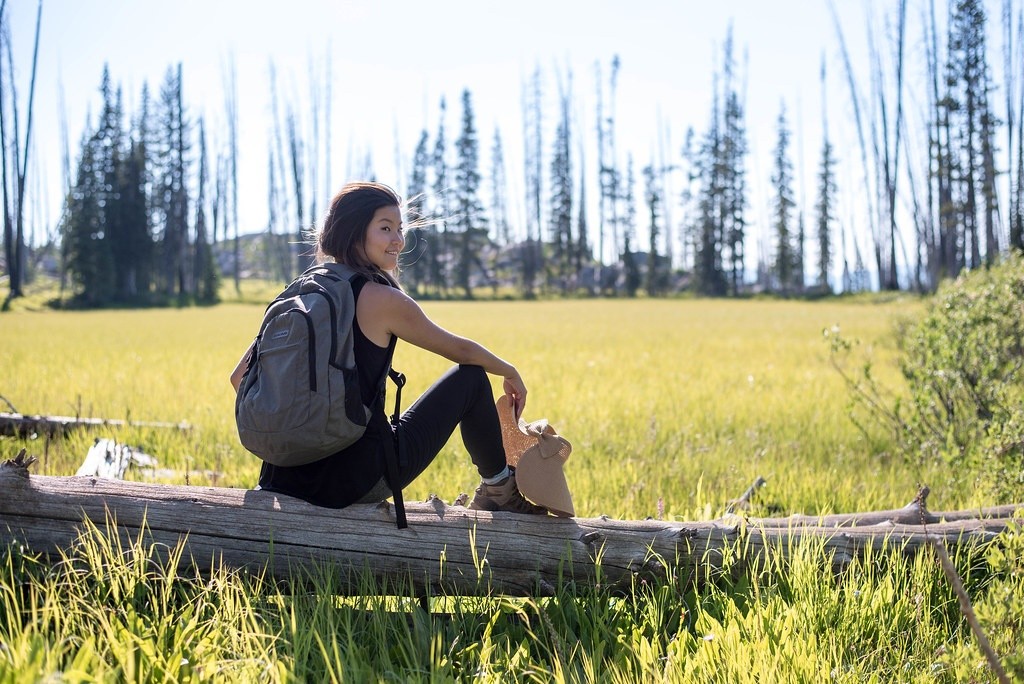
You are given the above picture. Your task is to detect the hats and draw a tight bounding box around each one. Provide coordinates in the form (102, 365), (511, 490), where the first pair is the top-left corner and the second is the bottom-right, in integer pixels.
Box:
(496, 395), (575, 518)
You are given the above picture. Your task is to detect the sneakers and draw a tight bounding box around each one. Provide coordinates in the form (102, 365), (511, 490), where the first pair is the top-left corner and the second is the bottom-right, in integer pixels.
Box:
(467, 464), (548, 516)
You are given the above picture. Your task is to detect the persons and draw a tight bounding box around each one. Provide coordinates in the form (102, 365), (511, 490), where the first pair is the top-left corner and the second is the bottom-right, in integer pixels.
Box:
(230, 183), (549, 515)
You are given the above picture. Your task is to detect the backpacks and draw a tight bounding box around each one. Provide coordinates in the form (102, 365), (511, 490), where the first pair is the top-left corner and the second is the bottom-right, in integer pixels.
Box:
(234, 261), (390, 467)
